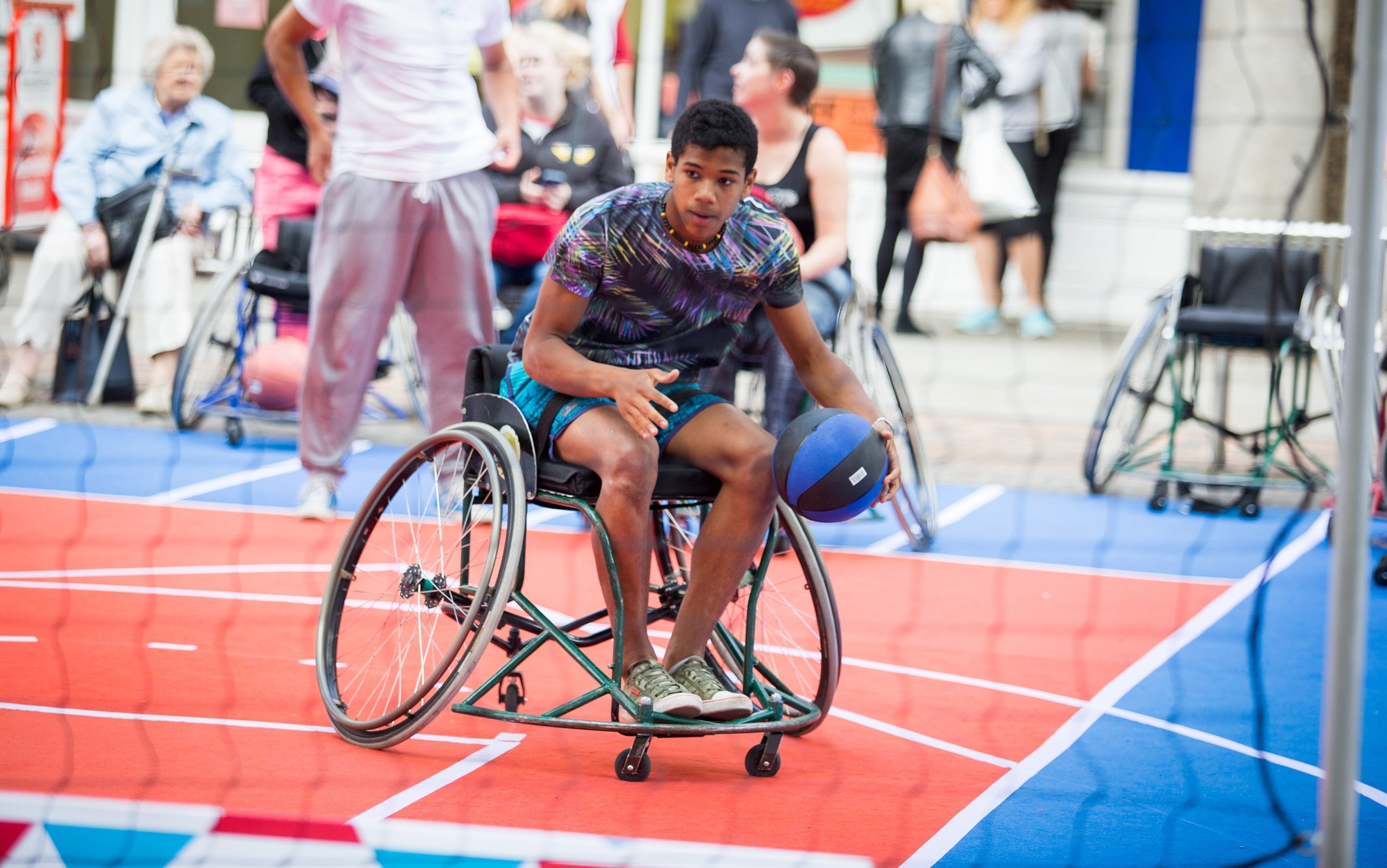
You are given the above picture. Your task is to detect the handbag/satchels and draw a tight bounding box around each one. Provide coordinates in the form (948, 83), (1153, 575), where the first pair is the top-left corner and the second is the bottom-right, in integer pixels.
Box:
(489, 200), (572, 260)
(95, 181), (170, 271)
(953, 120), (1041, 223)
(906, 156), (979, 248)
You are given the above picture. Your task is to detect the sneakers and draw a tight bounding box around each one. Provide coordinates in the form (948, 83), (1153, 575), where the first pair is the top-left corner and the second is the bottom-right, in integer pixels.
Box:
(669, 654), (753, 719)
(619, 659), (703, 723)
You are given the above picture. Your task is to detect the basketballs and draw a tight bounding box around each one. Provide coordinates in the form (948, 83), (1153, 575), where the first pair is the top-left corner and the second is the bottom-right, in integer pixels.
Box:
(771, 407), (890, 523)
(241, 336), (308, 409)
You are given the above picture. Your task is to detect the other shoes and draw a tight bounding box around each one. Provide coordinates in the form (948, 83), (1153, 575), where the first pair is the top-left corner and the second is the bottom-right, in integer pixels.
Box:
(893, 311), (922, 334)
(1017, 305), (1052, 339)
(302, 486), (336, 524)
(431, 491), (493, 522)
(136, 388), (172, 413)
(0, 371), (32, 408)
(957, 305), (1006, 336)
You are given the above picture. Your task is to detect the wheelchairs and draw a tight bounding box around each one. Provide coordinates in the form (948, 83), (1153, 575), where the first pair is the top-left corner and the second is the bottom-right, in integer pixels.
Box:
(1079, 241), (1335, 521)
(165, 219), (320, 449)
(737, 302), (952, 548)
(316, 347), (845, 790)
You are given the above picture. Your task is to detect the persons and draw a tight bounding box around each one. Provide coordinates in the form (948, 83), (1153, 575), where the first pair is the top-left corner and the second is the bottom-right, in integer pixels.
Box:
(499, 100), (901, 720)
(858, 0), (1107, 334)
(1, 0), (855, 524)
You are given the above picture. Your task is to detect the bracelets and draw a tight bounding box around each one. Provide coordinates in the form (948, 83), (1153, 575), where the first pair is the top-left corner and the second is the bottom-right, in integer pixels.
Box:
(870, 415), (897, 437)
